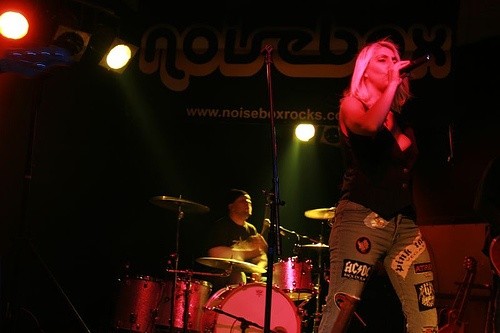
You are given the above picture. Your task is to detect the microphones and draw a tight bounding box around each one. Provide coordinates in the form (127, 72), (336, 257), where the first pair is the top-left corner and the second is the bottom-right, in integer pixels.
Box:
(399, 55), (431, 75)
(263, 219), (285, 236)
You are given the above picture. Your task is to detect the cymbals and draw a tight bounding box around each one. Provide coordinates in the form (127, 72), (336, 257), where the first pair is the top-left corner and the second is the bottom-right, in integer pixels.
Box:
(149, 195), (210, 214)
(302, 243), (330, 248)
(304, 208), (337, 221)
(195, 256), (268, 274)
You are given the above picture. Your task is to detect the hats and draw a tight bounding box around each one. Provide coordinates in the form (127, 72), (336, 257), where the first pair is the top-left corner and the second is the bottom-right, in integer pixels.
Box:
(225, 187), (247, 206)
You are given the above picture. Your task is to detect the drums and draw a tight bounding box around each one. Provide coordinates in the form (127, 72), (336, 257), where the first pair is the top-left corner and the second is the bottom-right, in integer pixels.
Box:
(154, 276), (213, 333)
(272, 254), (315, 302)
(109, 273), (157, 333)
(199, 283), (303, 333)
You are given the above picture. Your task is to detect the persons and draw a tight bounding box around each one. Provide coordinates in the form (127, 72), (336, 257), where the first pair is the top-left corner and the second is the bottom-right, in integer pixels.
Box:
(317, 38), (439, 333)
(200, 188), (269, 282)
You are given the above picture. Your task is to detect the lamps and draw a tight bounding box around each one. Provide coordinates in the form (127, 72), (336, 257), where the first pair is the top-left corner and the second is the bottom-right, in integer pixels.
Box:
(97, 37), (139, 75)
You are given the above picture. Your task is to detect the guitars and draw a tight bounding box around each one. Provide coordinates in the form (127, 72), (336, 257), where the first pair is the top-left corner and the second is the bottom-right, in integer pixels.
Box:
(438, 255), (477, 333)
